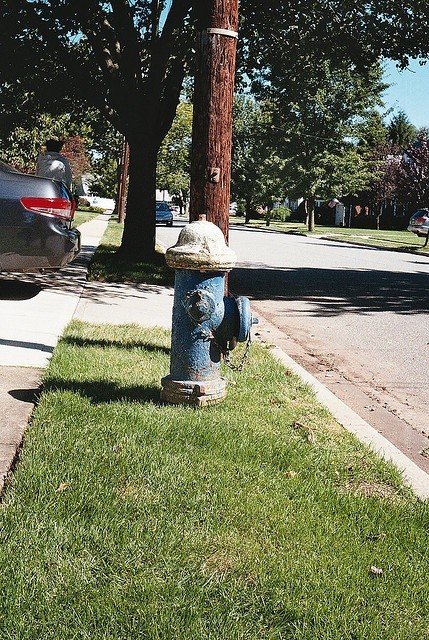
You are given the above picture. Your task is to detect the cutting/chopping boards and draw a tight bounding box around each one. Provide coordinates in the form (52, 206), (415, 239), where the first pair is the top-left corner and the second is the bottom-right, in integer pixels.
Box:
(159, 214), (259, 407)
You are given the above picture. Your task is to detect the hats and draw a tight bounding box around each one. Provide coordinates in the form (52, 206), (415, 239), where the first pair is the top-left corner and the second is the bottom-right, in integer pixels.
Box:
(0, 159), (81, 272)
(156, 202), (174, 225)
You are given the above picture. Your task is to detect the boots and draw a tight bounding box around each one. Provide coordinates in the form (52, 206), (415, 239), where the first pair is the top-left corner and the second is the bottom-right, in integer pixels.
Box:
(407, 208), (429, 237)
